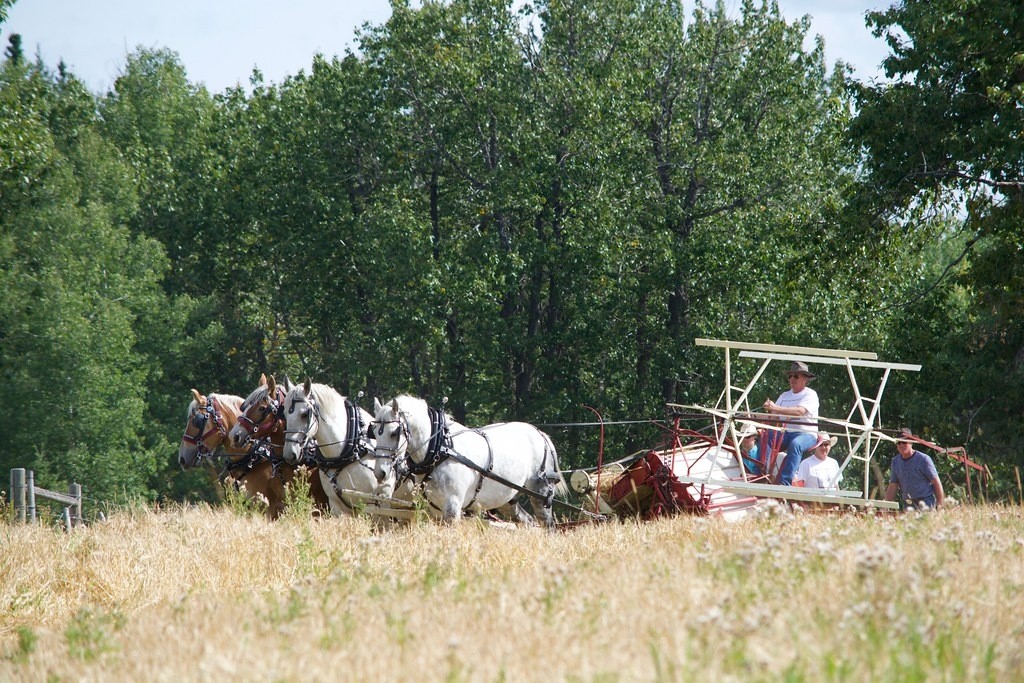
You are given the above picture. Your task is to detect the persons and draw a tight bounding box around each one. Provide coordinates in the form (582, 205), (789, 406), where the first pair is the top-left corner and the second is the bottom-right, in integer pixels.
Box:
(885, 428), (944, 512)
(754, 361), (818, 487)
(735, 425), (762, 476)
(795, 423), (845, 489)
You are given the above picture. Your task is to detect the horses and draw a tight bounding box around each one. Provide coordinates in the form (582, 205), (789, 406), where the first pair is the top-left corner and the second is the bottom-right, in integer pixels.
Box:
(177, 372), (574, 533)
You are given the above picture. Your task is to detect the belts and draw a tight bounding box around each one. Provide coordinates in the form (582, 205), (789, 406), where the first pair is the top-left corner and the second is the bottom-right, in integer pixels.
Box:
(903, 498), (926, 505)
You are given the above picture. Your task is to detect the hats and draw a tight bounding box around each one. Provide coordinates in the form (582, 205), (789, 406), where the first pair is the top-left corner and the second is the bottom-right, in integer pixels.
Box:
(808, 431), (838, 452)
(895, 428), (919, 440)
(735, 423), (759, 437)
(782, 362), (816, 380)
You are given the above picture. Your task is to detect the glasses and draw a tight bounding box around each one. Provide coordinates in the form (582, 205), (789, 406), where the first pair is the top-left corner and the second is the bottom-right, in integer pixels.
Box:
(745, 436), (752, 439)
(788, 374), (801, 379)
(897, 442), (908, 444)
(819, 443), (829, 447)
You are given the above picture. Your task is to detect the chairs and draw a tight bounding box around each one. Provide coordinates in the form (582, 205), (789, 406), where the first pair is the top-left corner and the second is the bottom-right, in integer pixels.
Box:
(769, 435), (825, 485)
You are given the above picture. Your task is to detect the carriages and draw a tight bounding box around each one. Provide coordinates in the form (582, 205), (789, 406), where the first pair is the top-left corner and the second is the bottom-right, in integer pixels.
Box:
(174, 336), (997, 533)
